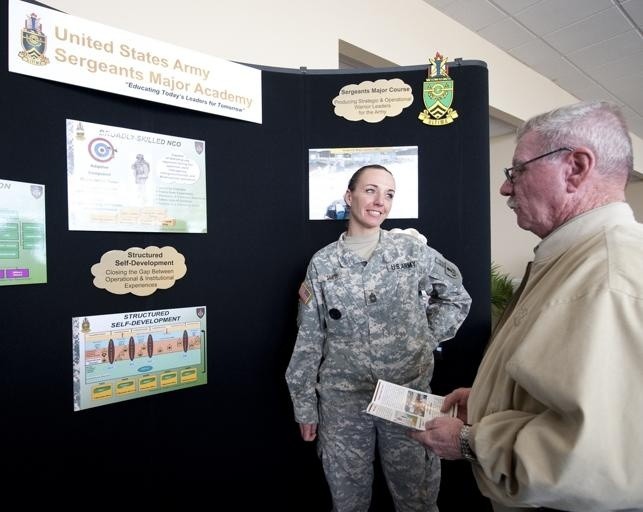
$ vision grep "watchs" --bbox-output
[459,424,476,463]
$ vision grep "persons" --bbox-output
[284,164,473,512]
[403,102,643,512]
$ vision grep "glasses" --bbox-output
[504,146,573,183]
